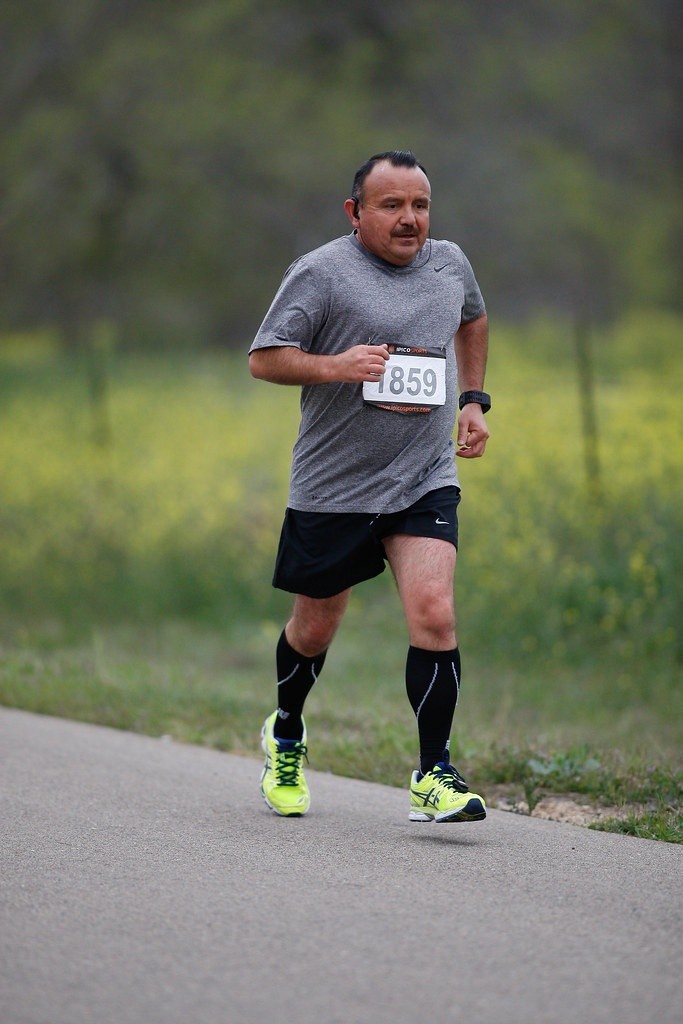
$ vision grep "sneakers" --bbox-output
[409,749,487,824]
[261,710,311,817]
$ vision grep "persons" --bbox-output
[245,151,491,828]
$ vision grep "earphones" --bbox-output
[351,196,360,219]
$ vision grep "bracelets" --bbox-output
[458,391,492,414]
